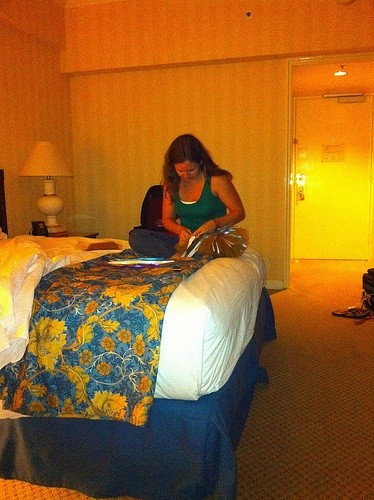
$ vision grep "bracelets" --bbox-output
[213,219,221,228]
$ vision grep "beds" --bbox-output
[0,169,277,500]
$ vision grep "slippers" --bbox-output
[333,306,354,315]
[347,309,370,318]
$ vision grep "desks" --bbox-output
[68,232,99,239]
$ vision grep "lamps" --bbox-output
[17,142,73,237]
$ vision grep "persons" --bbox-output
[129,134,248,261]
